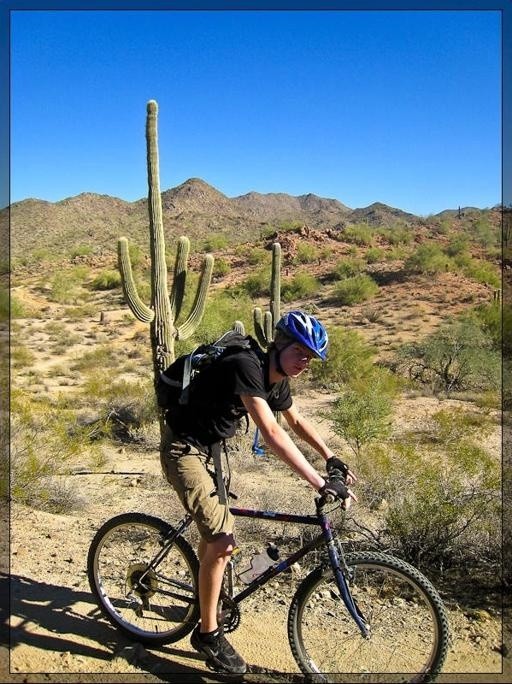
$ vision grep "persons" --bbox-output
[158,311,358,678]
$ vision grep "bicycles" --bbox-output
[87,459,452,684]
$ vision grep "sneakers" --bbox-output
[190,622,248,677]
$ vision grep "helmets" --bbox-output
[275,310,328,362]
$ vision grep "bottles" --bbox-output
[236,546,279,586]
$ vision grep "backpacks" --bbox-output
[154,332,266,413]
